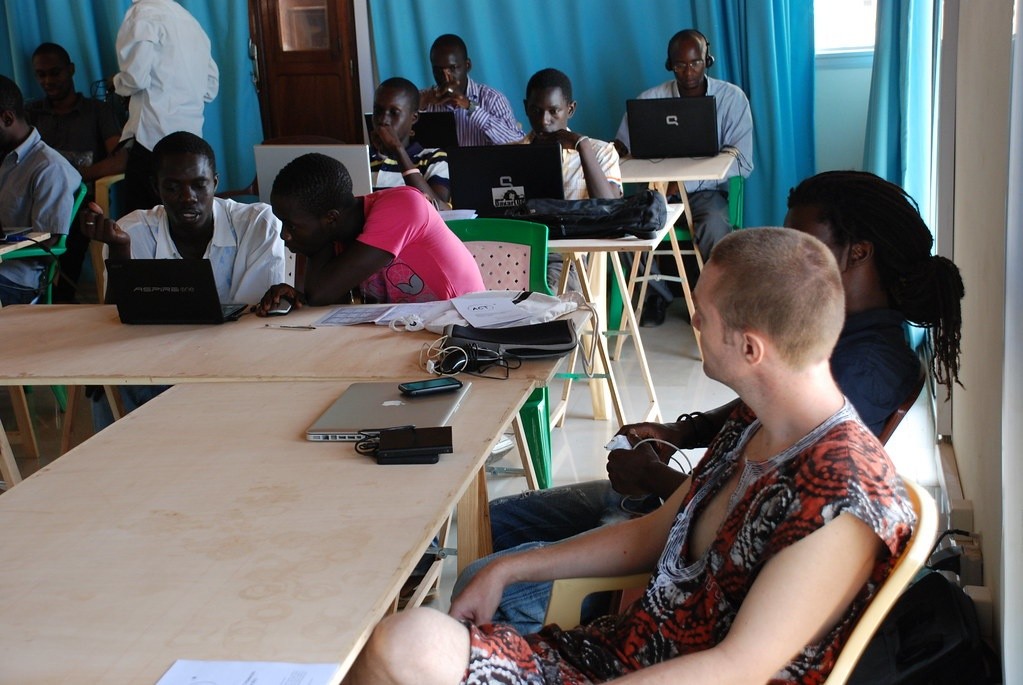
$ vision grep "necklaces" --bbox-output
[349,280,366,304]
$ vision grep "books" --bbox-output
[318,304,454,325]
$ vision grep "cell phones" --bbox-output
[398,377,464,396]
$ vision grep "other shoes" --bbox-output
[484,433,515,465]
[647,294,667,325]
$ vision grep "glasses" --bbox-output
[34,70,68,79]
[670,60,707,73]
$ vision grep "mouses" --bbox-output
[440,348,500,373]
[267,295,292,316]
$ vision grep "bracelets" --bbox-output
[402,169,420,176]
[677,413,699,449]
[690,411,714,441]
[575,136,588,150]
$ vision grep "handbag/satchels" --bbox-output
[844,572,1002,685]
[503,188,667,240]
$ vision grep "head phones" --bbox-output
[665,29,715,71]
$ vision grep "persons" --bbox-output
[24,42,133,294]
[0,75,92,305]
[342,227,917,685]
[399,170,965,638]
[419,33,525,146]
[79,132,296,304]
[614,29,755,327]
[516,69,624,295]
[257,153,486,315]
[371,78,452,210]
[107,0,219,214]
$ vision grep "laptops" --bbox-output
[365,110,460,156]
[305,381,472,441]
[253,143,372,204]
[626,95,722,158]
[0,220,34,243]
[105,259,247,325]
[449,142,565,219]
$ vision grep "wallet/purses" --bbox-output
[442,318,578,359]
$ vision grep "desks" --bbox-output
[0,138,742,685]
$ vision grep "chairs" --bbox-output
[607,171,744,331]
[443,215,558,492]
[0,179,90,414]
[89,172,127,303]
[878,345,927,446]
[540,476,940,685]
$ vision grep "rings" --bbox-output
[86,222,94,225]
[448,88,453,93]
